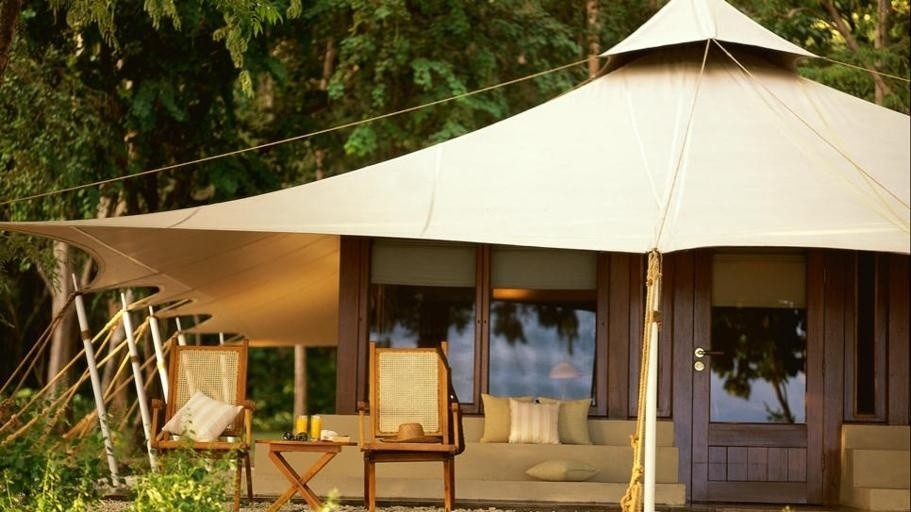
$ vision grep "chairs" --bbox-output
[359,336,464,512]
[147,334,255,510]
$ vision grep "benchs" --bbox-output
[835,422,911,511]
[236,395,687,508]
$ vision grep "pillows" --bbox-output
[560,395,596,446]
[509,398,560,442]
[162,389,243,442]
[524,458,600,483]
[478,393,510,442]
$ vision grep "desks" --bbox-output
[256,431,358,510]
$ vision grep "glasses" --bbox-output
[281,429,309,442]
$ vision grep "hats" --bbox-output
[379,421,441,444]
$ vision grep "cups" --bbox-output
[310,415,322,441]
[294,414,308,437]
